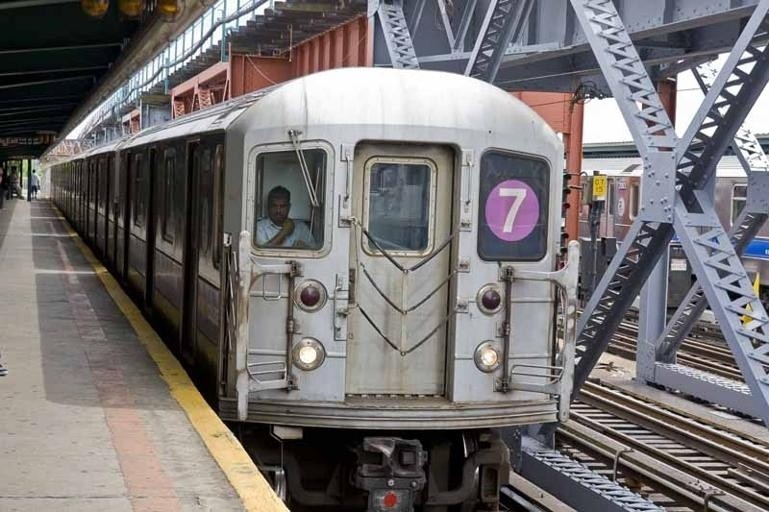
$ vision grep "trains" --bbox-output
[50,67,579,512]
[581,155,769,313]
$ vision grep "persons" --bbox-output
[252,184,318,251]
[31,169,43,201]
[9,165,25,199]
